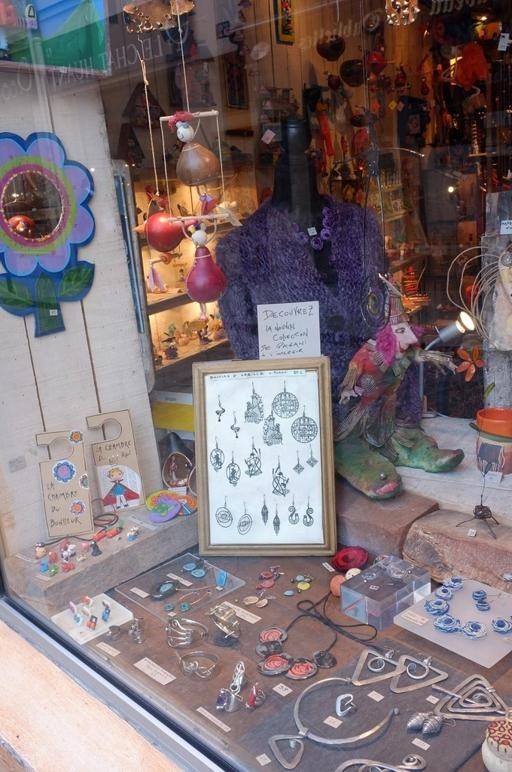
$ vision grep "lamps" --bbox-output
[412,311,477,416]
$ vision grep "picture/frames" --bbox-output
[191,356,338,558]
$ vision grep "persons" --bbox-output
[208,115,426,428]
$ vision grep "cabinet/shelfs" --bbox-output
[0,0,512,391]
